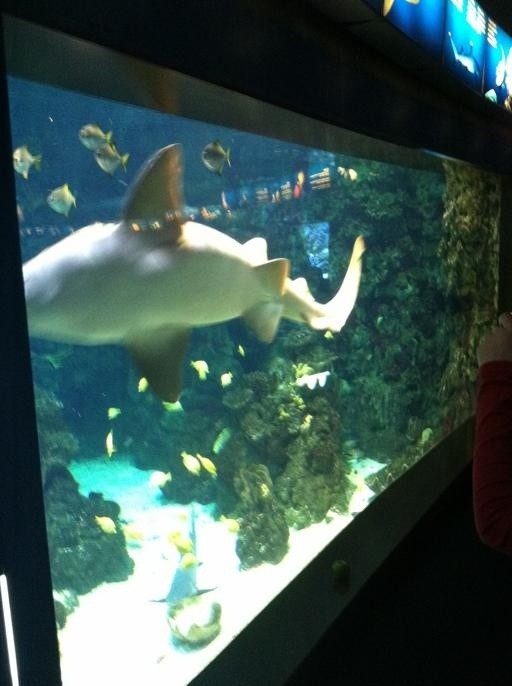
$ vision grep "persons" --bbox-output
[471,310,512,562]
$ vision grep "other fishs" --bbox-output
[10,119,367,655]
[420,426,434,446]
